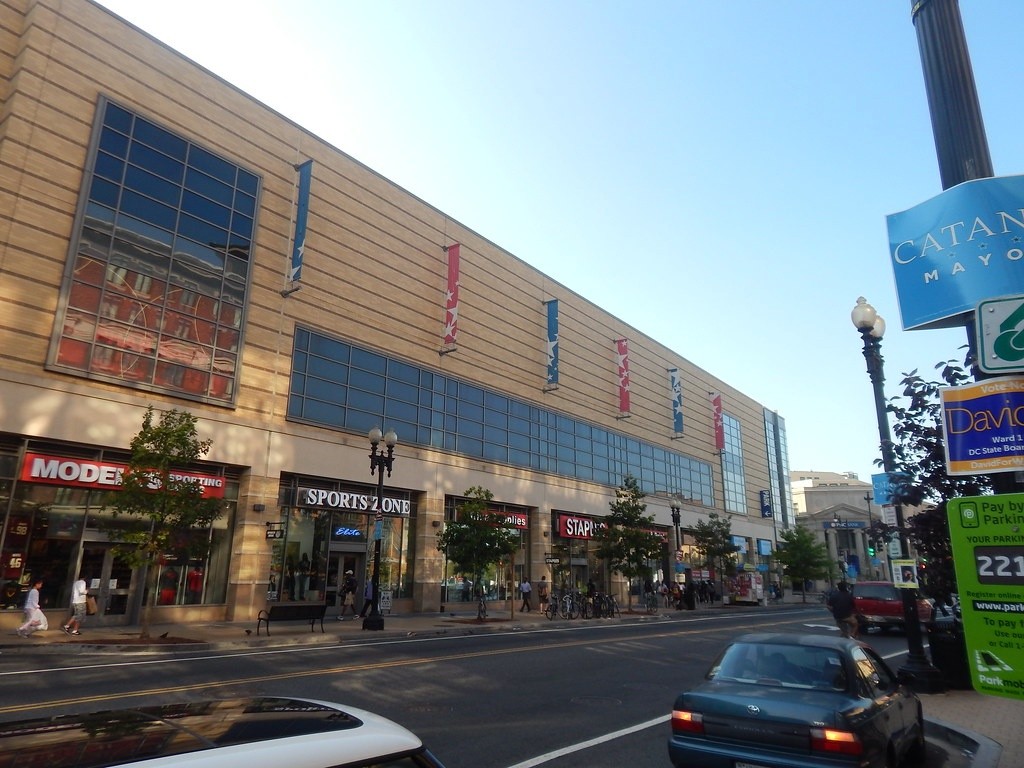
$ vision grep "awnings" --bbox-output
[737,562,769,572]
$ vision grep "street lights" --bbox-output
[849,294,945,694]
[360,422,398,632]
[668,494,682,550]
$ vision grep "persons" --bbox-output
[827,581,864,638]
[187,567,203,603]
[901,566,915,583]
[520,577,532,612]
[587,580,595,603]
[461,574,485,601]
[16,579,43,638]
[538,576,549,614]
[60,574,88,636]
[336,570,360,620]
[160,567,178,605]
[360,575,372,617]
[637,579,714,610]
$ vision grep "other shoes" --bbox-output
[352,615,361,620]
[336,616,344,621]
[71,631,82,636]
[360,614,367,618]
[16,627,30,639]
[527,608,531,612]
[540,610,547,615]
[60,626,71,636]
[848,634,856,640]
[520,610,523,612]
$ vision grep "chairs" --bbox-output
[767,652,806,683]
[820,659,845,687]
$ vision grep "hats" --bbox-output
[345,570,354,575]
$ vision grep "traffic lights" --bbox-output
[868,540,875,557]
[919,562,926,571]
[877,540,884,552]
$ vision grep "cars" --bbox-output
[0,693,450,768]
[851,579,935,636]
[665,629,926,768]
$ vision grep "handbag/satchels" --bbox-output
[34,606,48,631]
[86,591,98,615]
[344,591,354,606]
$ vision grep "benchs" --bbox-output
[257,605,327,637]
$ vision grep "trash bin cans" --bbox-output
[679,590,688,610]
[926,621,973,690]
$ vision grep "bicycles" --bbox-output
[647,593,660,615]
[473,593,489,622]
[544,590,622,621]
